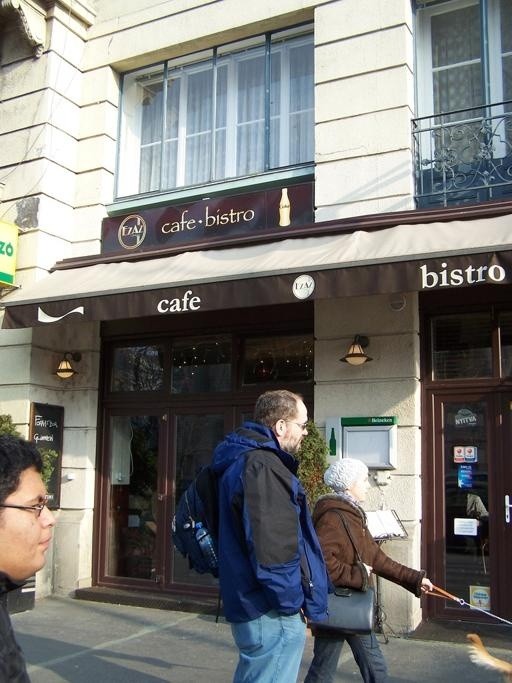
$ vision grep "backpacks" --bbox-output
[170,461,218,579]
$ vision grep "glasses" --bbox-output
[0,496,48,517]
[285,420,309,430]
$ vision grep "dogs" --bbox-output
[466,633,512,682]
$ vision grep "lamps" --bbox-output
[340,334,371,367]
[52,349,82,378]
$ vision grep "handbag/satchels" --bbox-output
[307,589,375,633]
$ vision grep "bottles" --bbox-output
[195,522,221,570]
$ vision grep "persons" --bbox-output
[303,458,434,682]
[213,389,329,682]
[1,432,57,683]
